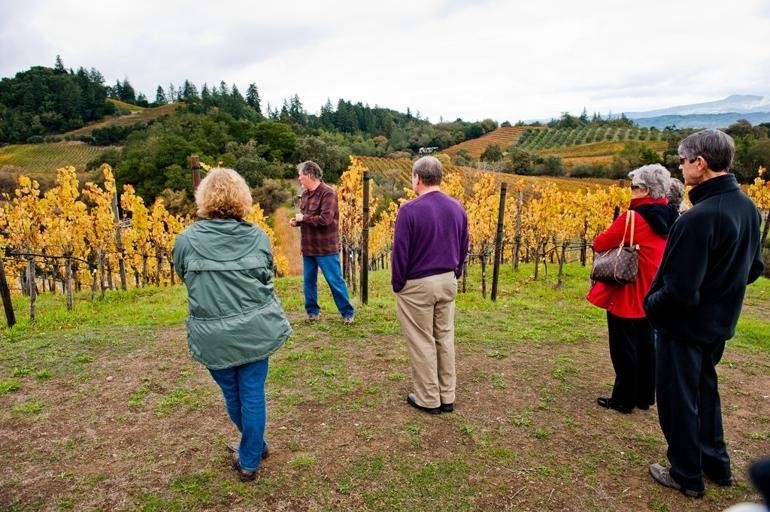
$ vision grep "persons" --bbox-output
[390,155,470,415]
[288,160,355,327]
[642,127,765,501]
[584,162,681,415]
[171,166,294,481]
[649,177,689,408]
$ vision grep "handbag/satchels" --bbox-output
[590,210,641,283]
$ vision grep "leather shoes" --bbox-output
[441,404,454,412]
[406,392,442,414]
[712,474,734,485]
[597,397,613,408]
[650,463,706,498]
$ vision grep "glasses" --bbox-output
[680,157,687,164]
[631,183,640,190]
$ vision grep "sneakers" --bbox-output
[310,314,319,321]
[231,451,257,481]
[343,314,355,325]
[227,440,270,458]
